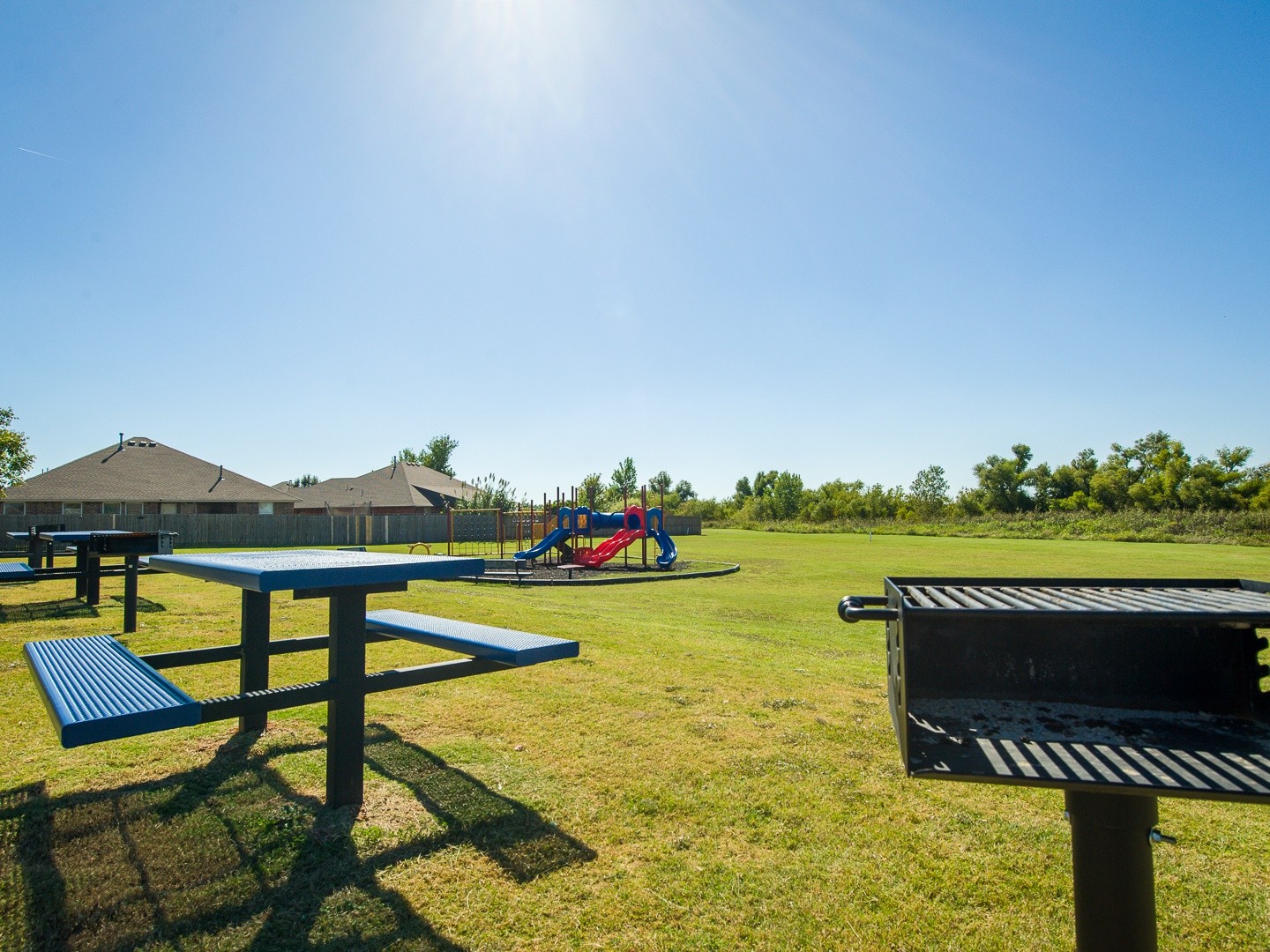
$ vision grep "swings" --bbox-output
[472,513,480,555]
[484,513,492,555]
[458,513,467,556]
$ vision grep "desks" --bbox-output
[148,548,487,810]
[39,530,134,604]
[6,531,36,568]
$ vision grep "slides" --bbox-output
[580,528,644,567]
[513,528,569,560]
[650,528,678,566]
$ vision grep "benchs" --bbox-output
[0,563,35,579]
[475,559,533,588]
[364,609,580,668]
[22,634,202,750]
[138,555,151,567]
[65,546,77,553]
